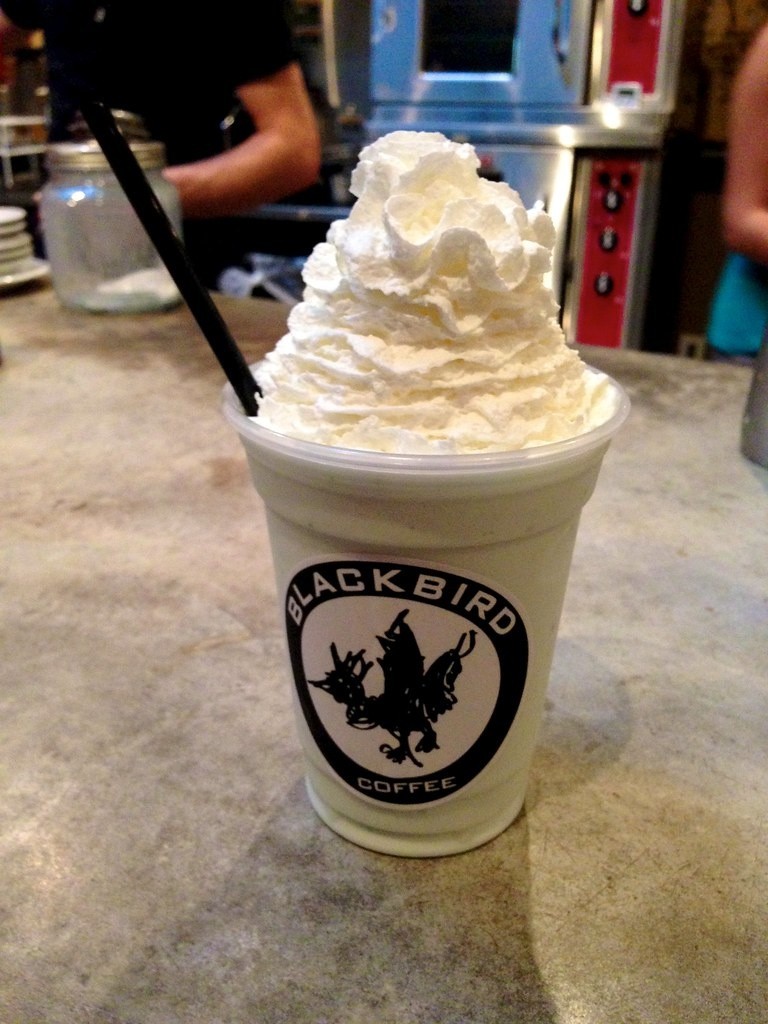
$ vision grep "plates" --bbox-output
[0,204,53,295]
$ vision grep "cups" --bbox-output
[218,368,628,859]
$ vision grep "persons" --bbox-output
[703,0,768,369]
[31,0,322,298]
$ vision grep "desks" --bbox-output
[1,278,767,1024]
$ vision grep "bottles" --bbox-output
[42,142,183,311]
